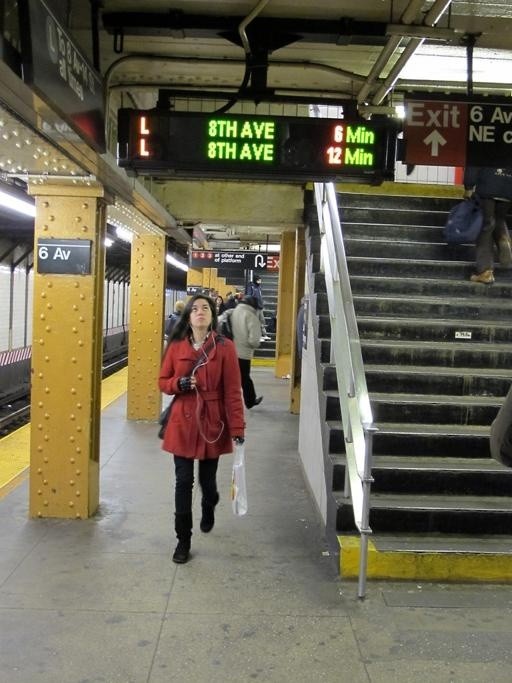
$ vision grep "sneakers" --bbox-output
[471,270,495,284]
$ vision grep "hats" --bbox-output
[239,295,262,309]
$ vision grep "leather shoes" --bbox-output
[248,396,262,408]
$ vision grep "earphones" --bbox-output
[188,320,191,325]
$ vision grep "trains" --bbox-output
[0,174,187,414]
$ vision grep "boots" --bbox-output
[173,513,192,563]
[201,495,218,531]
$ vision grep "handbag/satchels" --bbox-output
[232,447,249,516]
[159,405,171,439]
[445,203,482,242]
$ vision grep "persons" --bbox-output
[489,382,511,473]
[161,273,271,410]
[158,294,245,565]
[462,166,511,284]
